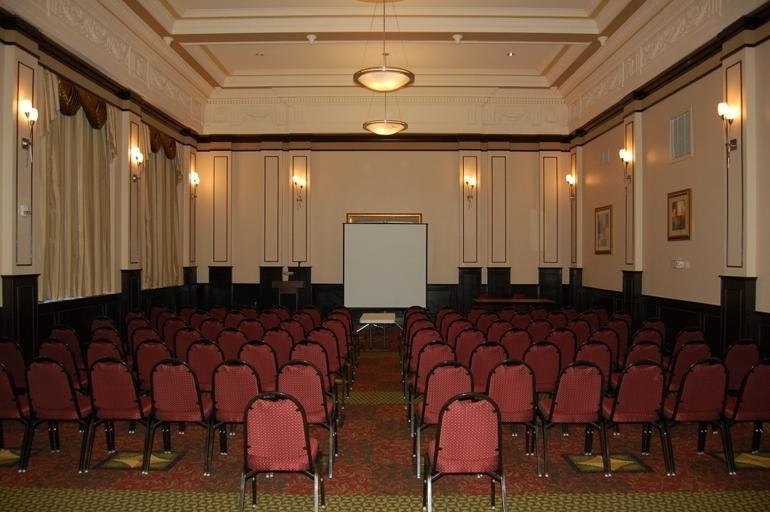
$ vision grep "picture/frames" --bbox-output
[595,205,613,255]
[667,188,692,241]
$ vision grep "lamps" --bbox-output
[191,172,200,200]
[292,176,306,201]
[717,102,738,151]
[363,53,408,137]
[133,148,144,182]
[353,1,415,94]
[465,176,477,201]
[619,149,632,183]
[566,174,576,199]
[22,99,38,149]
